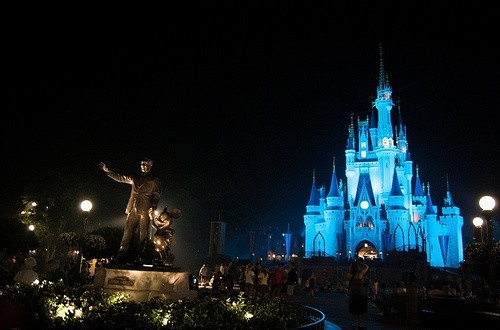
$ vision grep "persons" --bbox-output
[211,260,318,299]
[199,264,208,284]
[372,274,477,313]
[14,256,40,287]
[99,158,160,264]
[348,262,369,330]
[48,259,90,287]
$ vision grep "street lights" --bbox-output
[68,200,95,291]
[479,196,496,245]
[472,215,484,244]
